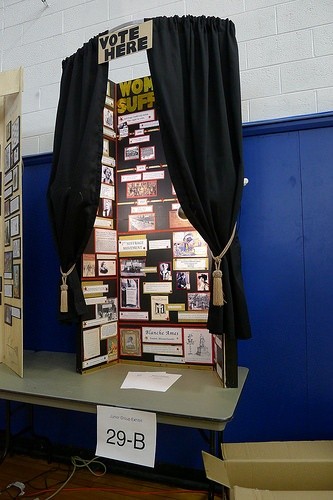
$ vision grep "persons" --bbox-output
[81,165,209,320]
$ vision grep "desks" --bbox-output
[0,350,249,500]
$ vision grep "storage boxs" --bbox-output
[202,440,333,500]
